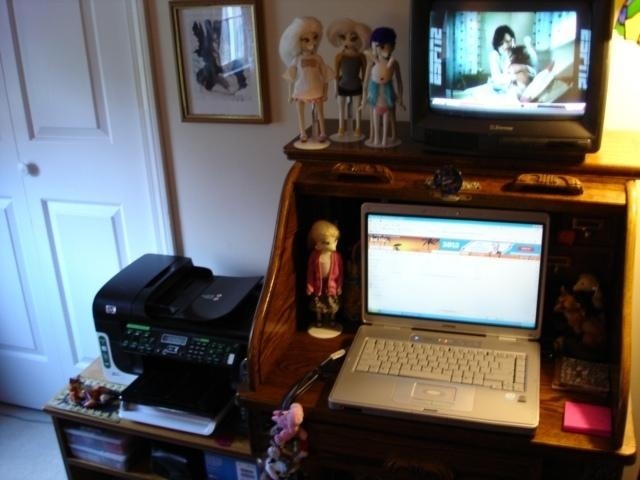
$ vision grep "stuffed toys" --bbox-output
[551,223,606,352]
[260,403,312,480]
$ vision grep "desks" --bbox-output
[244,117,639,478]
[42,353,252,480]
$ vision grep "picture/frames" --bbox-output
[168,0,272,124]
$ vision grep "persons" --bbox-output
[460,23,536,105]
[356,26,406,142]
[67,379,121,412]
[497,43,536,97]
[368,47,397,147]
[278,15,333,142]
[325,16,373,138]
[305,220,345,328]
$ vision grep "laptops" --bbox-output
[324,201,551,435]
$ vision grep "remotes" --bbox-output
[515,173,583,194]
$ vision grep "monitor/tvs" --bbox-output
[408,0,612,168]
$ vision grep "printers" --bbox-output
[91,253,267,385]
